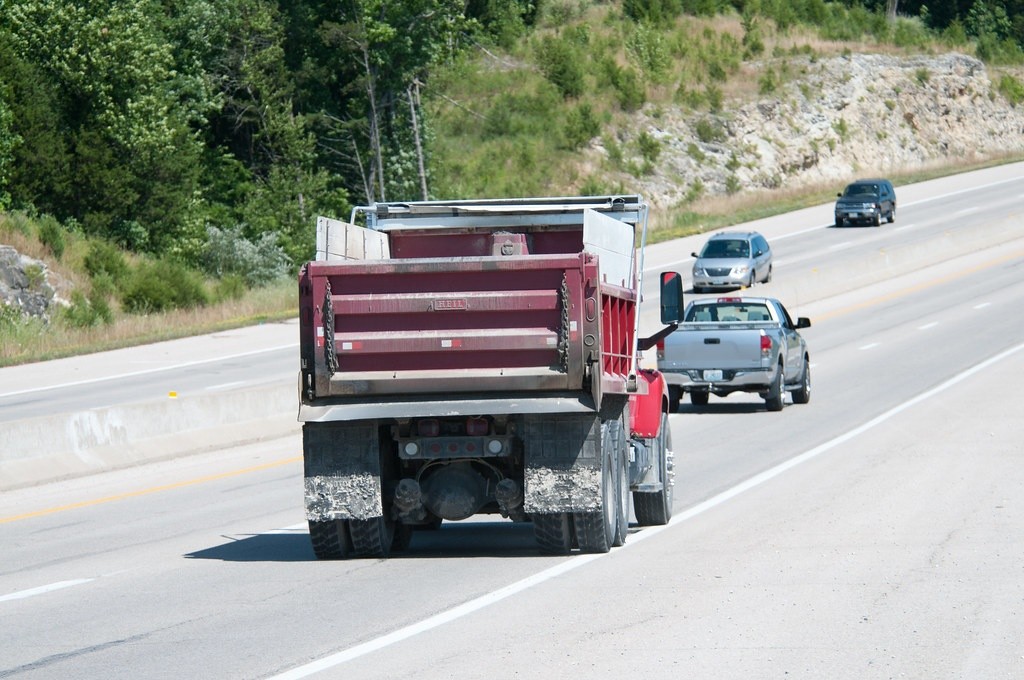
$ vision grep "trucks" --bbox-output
[297,187,685,560]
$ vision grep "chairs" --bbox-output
[748,312,764,320]
[696,312,711,321]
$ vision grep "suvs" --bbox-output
[836,179,897,228]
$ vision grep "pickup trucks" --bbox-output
[657,297,815,411]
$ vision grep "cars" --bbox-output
[692,230,773,292]
